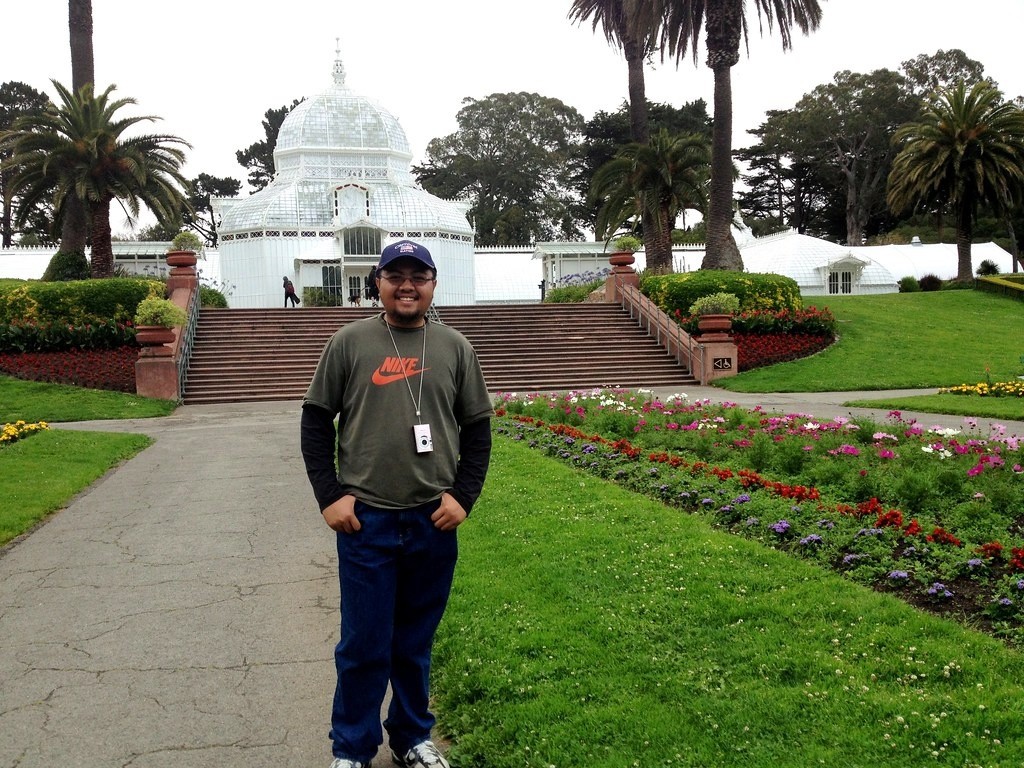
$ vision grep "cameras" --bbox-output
[413,423,434,453]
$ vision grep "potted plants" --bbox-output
[608,235,643,274]
[688,291,740,341]
[166,232,204,276]
[133,296,189,356]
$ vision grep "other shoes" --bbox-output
[372,302,378,307]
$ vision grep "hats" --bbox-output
[375,239,437,277]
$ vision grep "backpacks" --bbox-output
[286,284,294,296]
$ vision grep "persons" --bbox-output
[366,265,380,308]
[283,276,295,308]
[300,239,498,768]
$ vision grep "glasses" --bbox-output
[378,272,432,286]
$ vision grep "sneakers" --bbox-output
[391,741,450,768]
[328,757,372,768]
